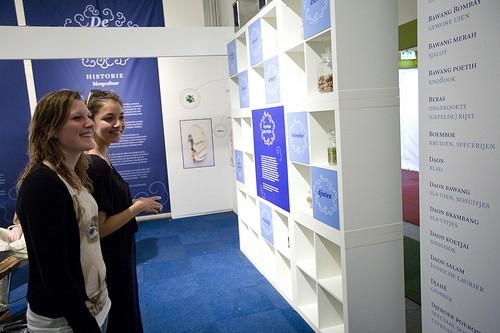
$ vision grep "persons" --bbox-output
[15,89,111,333]
[85,89,163,333]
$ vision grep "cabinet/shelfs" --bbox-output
[223,0,408,333]
